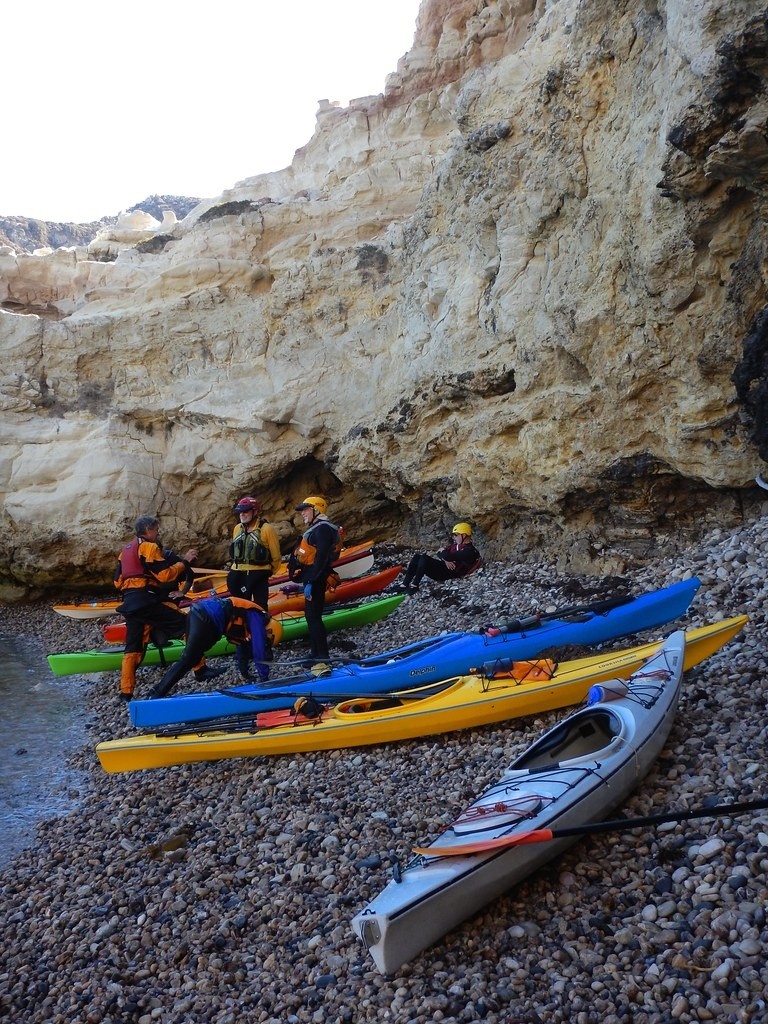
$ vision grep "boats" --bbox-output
[51,539,408,641]
[128,575,716,729]
[349,626,684,976]
[46,588,413,677]
[91,614,751,773]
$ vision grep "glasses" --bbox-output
[239,508,252,514]
[454,533,460,537]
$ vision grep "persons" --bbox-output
[113,515,229,704]
[225,497,282,683]
[389,522,483,598]
[287,496,345,679]
[147,596,284,703]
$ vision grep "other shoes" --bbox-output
[119,692,134,703]
[391,583,420,595]
[194,665,228,682]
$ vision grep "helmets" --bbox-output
[235,496,260,521]
[452,522,472,537]
[294,496,328,515]
[265,618,284,648]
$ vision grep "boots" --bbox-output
[148,671,180,699]
[287,632,332,668]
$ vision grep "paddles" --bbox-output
[256,657,376,667]
[412,798,767,857]
[213,688,438,702]
